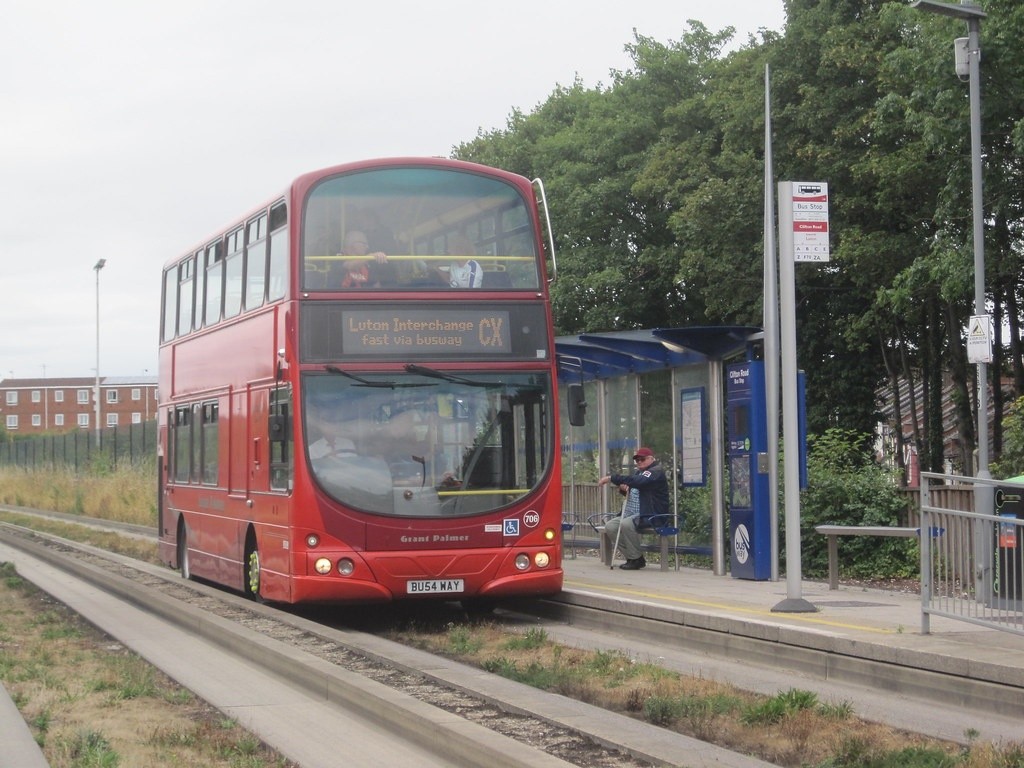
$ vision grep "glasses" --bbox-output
[636,457,651,463]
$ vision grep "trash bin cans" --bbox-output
[996,485,1024,600]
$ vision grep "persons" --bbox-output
[307,407,455,486]
[598,448,670,570]
[327,229,481,290]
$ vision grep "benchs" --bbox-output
[561,512,579,560]
[814,524,944,600]
[586,512,686,572]
[480,263,513,288]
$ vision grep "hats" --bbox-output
[632,447,654,459]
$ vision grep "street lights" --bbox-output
[909,0,994,608]
[92,260,106,453]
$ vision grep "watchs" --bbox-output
[608,475,612,482]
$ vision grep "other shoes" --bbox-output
[620,557,646,569]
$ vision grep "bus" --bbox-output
[156,157,588,614]
